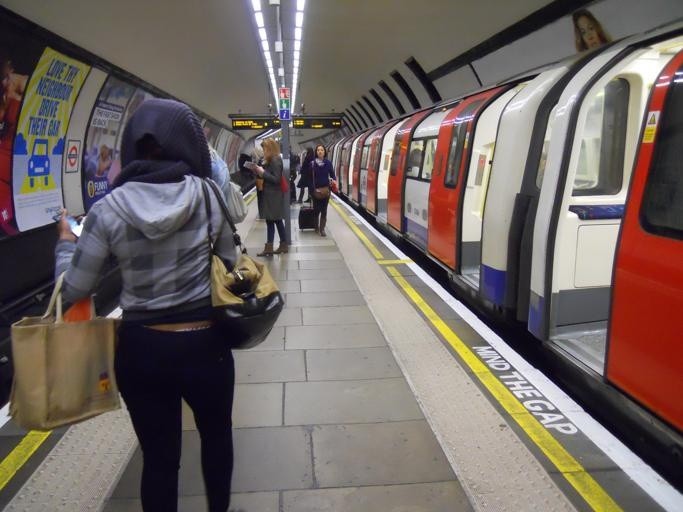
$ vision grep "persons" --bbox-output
[206,139,230,196]
[54,99,234,511]
[253,138,336,256]
[573,8,611,53]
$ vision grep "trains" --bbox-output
[325,13,683,481]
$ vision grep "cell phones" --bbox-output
[51,211,83,237]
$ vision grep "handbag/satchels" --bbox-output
[281,175,289,193]
[313,187,329,200]
[201,177,284,349]
[7,271,122,432]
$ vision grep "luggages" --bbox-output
[299,201,315,232]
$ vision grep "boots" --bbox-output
[314,215,318,233]
[320,217,326,235]
[256,242,273,257]
[273,242,288,254]
